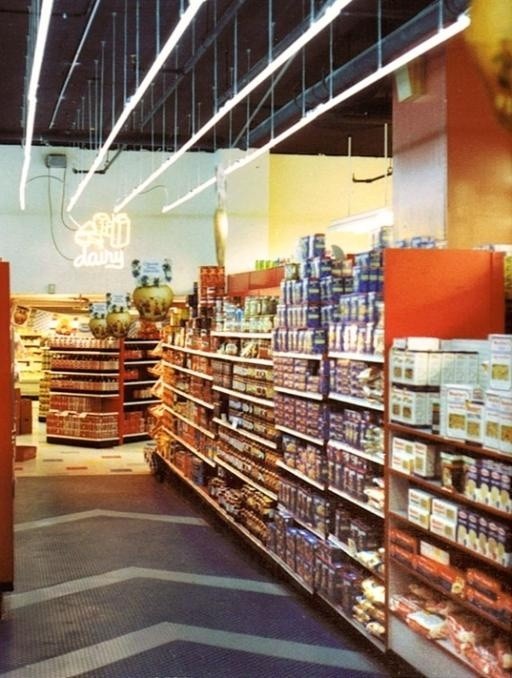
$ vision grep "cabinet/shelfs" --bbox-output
[46,336,122,445]
[213,326,277,548]
[269,350,324,605]
[317,349,387,656]
[154,330,213,509]
[122,336,160,448]
[387,335,512,678]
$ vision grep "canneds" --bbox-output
[270,224,392,354]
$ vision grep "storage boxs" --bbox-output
[13,389,21,435]
[20,399,32,433]
